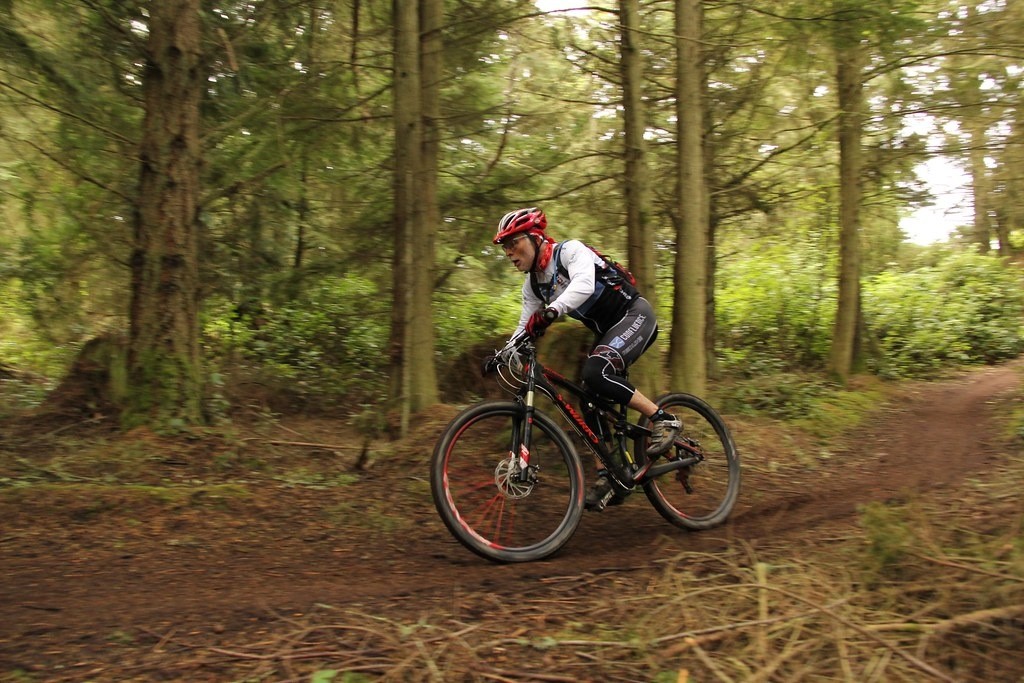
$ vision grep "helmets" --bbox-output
[493,207,547,244]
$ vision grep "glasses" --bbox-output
[502,235,528,251]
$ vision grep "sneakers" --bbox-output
[646,414,684,454]
[585,477,611,504]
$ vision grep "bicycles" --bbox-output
[430,307,741,563]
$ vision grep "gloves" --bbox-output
[525,311,544,336]
[482,356,500,376]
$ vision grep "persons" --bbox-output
[480,206,684,509]
